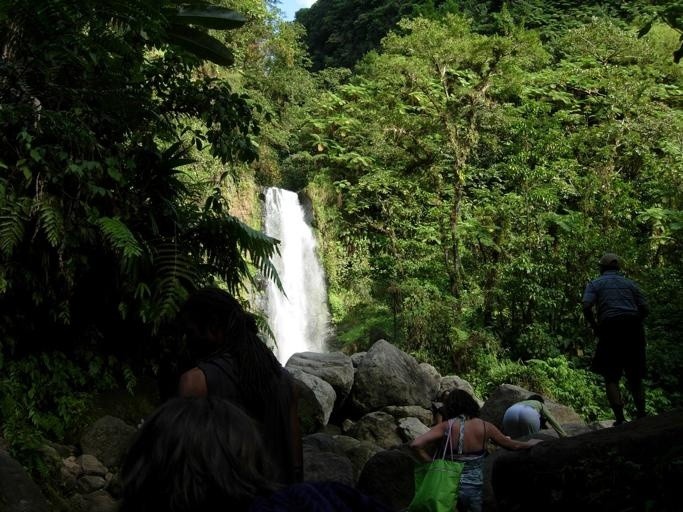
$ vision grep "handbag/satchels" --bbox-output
[407,460,465,511]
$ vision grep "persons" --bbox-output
[410,389,543,512]
[178,285,305,486]
[101,392,388,511]
[498,394,569,441]
[582,254,651,427]
